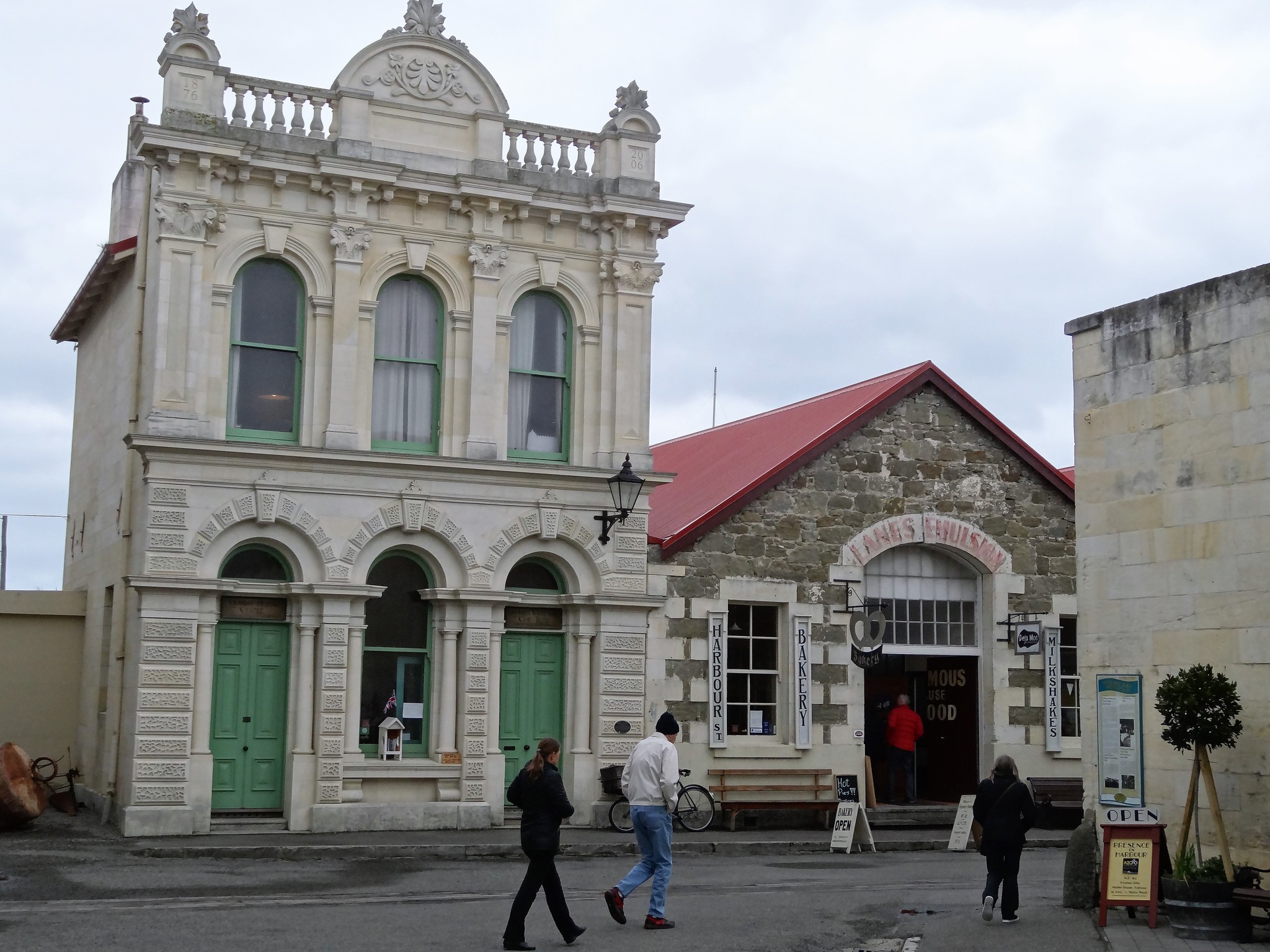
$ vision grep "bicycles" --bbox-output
[608,768,715,833]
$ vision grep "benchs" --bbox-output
[707,769,839,832]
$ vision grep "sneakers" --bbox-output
[1002,915,1019,922]
[981,896,993,921]
[643,914,675,929]
[604,888,628,925]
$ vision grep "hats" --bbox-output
[656,712,680,734]
[898,695,909,705]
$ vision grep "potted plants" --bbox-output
[1154,662,1258,942]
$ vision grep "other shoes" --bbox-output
[503,940,536,951]
[565,927,586,944]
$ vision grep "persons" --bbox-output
[604,712,679,929]
[972,754,1036,923]
[884,694,924,804]
[502,737,587,951]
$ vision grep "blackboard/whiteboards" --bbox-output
[834,773,861,803]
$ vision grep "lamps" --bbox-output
[593,453,646,545]
[728,618,742,630]
[259,385,290,401]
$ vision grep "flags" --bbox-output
[383,691,396,714]
